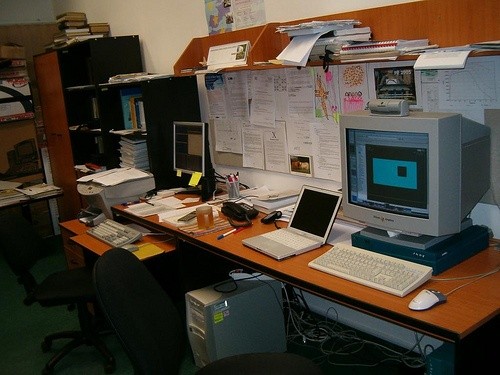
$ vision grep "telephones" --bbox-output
[221,202,259,226]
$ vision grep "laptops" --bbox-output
[240,182,344,263]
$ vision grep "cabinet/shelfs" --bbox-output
[168,0,500,78]
[32,35,143,270]
[0,44,45,183]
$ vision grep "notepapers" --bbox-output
[177,170,181,177]
[188,171,203,187]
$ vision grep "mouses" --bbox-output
[407,287,448,314]
[120,243,141,253]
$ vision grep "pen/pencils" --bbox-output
[225,172,240,198]
[218,227,236,240]
[234,227,247,234]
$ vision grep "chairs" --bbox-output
[0,206,119,374]
[93,248,320,374]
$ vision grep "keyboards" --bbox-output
[85,217,142,250]
[307,240,435,299]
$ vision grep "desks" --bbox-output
[57,183,500,342]
[0,182,65,206]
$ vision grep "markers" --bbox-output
[122,199,143,205]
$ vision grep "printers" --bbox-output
[76,165,156,227]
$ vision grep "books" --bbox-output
[340,39,440,61]
[253,190,300,214]
[46,11,103,49]
[275,21,371,61]
[119,89,148,132]
[89,21,110,37]
[85,163,103,172]
[0,183,63,208]
[118,137,150,172]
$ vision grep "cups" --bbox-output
[196,205,214,230]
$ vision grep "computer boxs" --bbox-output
[350,223,490,277]
[423,341,454,375]
[184,270,291,369]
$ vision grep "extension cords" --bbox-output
[228,267,277,282]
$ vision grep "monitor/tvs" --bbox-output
[171,118,224,204]
[337,107,493,252]
